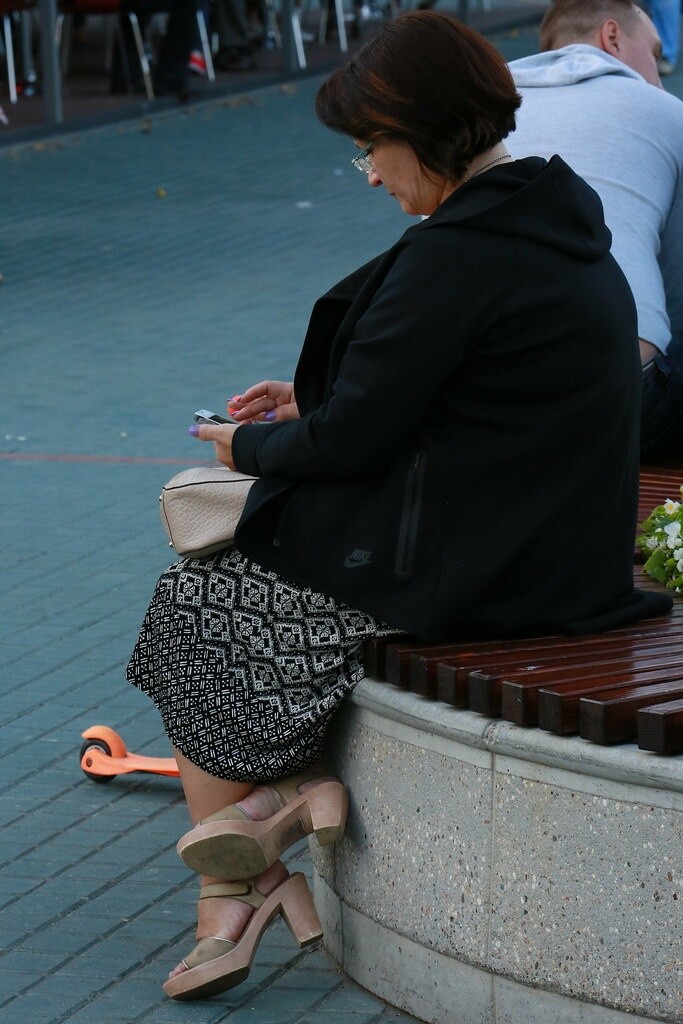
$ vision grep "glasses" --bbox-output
[351,142,373,174]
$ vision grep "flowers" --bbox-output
[635,485,683,593]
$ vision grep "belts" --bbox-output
[642,351,672,384]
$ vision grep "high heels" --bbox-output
[176,769,350,881]
[162,871,324,1001]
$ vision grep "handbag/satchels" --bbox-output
[158,466,260,559]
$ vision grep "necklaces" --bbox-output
[464,155,512,182]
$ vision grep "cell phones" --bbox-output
[193,409,238,425]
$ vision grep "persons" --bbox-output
[110,1,268,96]
[503,0,683,467]
[126,9,673,998]
[314,0,363,41]
[640,0,680,72]
